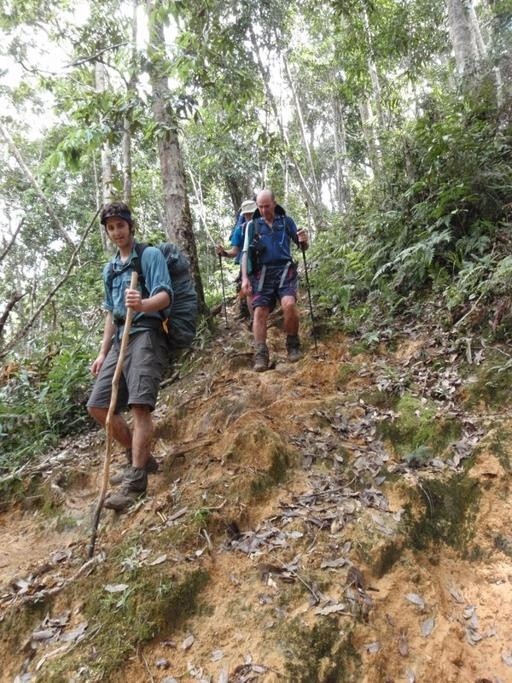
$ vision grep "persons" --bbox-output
[85,200,174,511]
[214,199,258,326]
[240,189,310,373]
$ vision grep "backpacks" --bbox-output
[106,242,199,350]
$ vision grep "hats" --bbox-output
[239,200,258,217]
[252,204,286,220]
[100,209,132,226]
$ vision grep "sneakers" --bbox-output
[233,311,249,320]
[103,467,148,510]
[108,445,159,486]
[285,334,304,363]
[253,343,269,372]
